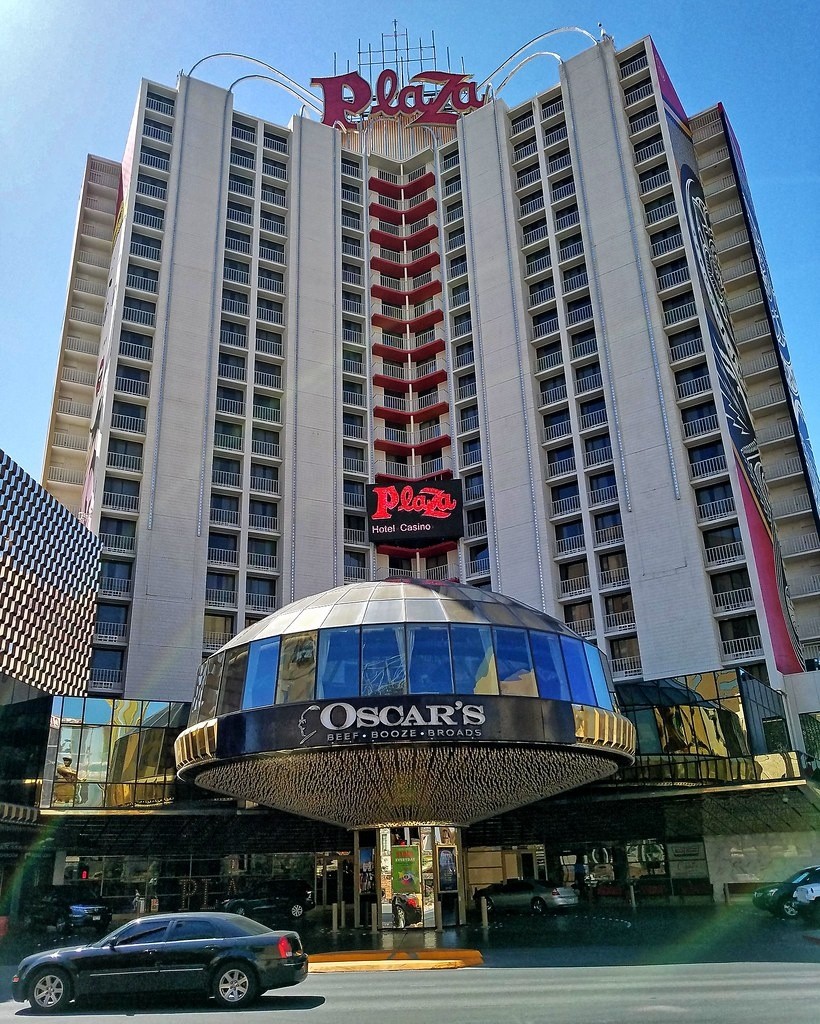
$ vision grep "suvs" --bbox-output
[18,884,113,936]
[220,878,317,923]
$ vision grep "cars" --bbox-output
[473,878,579,918]
[752,864,820,920]
[12,912,309,1016]
